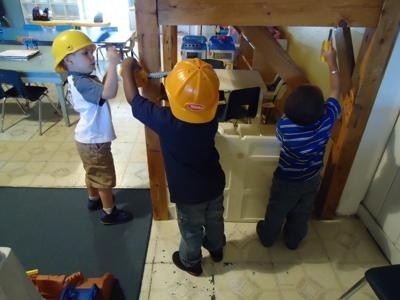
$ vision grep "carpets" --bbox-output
[0,186,153,300]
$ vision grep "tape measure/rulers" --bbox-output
[319,29,332,62]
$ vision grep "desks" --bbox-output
[0,45,73,127]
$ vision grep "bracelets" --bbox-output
[330,69,339,74]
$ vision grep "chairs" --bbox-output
[204,59,287,123]
[337,263,399,300]
[0,69,58,136]
[0,23,137,65]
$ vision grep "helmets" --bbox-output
[164,58,220,124]
[52,29,96,73]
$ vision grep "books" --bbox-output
[0,49,40,62]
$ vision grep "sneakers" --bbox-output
[257,220,272,247]
[283,221,298,250]
[87,193,116,211]
[172,251,202,276]
[99,205,132,225]
[202,234,223,262]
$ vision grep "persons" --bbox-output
[52,29,133,225]
[121,58,226,276]
[257,38,342,249]
